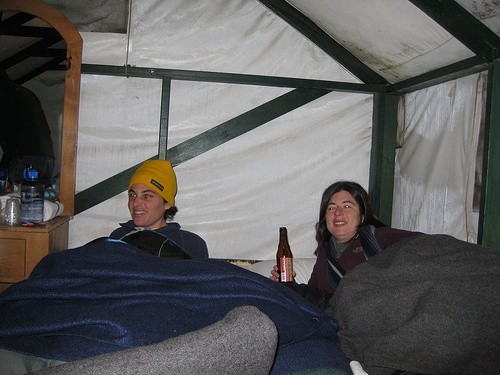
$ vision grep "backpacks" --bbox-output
[0,67,56,189]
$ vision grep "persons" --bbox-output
[271,180,428,311]
[0,66,56,189]
[107,159,210,259]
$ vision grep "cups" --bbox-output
[4,198,20,225]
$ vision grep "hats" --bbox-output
[128,159,177,208]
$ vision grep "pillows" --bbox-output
[246,257,316,285]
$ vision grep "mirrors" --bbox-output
[0,0,83,219]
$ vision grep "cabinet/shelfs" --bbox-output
[0,216,70,284]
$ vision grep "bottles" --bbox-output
[277,227,294,284]
[21,168,44,223]
